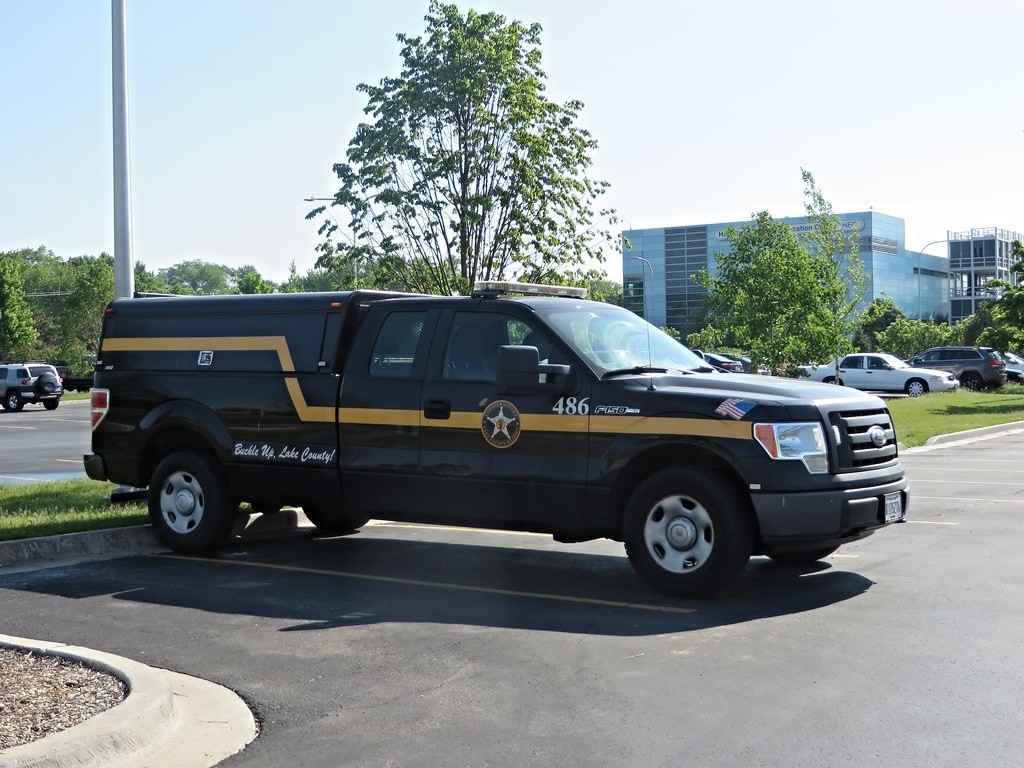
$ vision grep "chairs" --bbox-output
[522,333,549,362]
[459,318,508,370]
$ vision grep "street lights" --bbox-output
[916,239,962,326]
[630,255,657,327]
[302,196,358,288]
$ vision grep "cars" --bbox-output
[581,349,788,378]
[997,349,1024,382]
[799,352,961,397]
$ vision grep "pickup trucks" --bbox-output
[81,279,912,599]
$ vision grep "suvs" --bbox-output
[899,345,1008,391]
[0,362,65,415]
